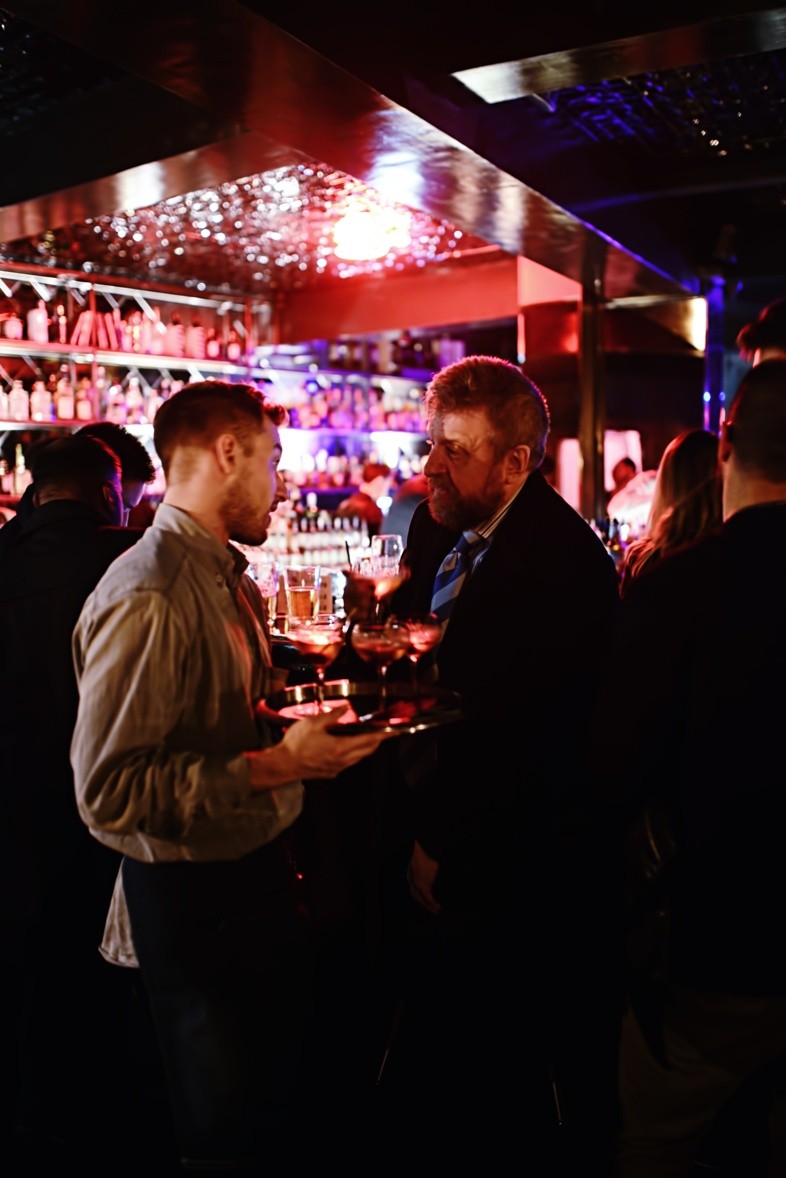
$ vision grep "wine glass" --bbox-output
[290,613,345,715]
[352,622,412,728]
[386,613,442,727]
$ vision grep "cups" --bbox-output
[247,565,322,634]
[370,531,403,582]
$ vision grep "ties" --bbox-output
[429,532,490,680]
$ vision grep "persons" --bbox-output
[615,427,725,588]
[380,435,437,557]
[74,423,159,530]
[605,456,637,504]
[336,459,395,535]
[66,379,394,1177]
[0,434,153,1178]
[608,352,786,1177]
[324,349,634,1178]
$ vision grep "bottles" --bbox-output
[608,518,624,562]
[235,512,369,569]
[1,293,204,359]
[0,374,185,423]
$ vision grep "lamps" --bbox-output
[332,206,395,262]
